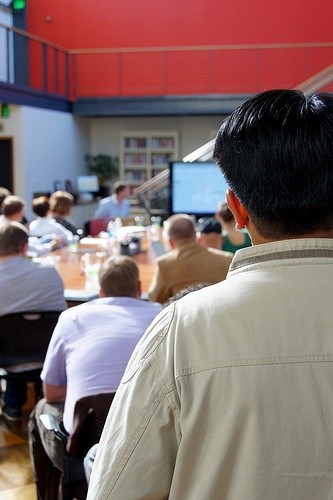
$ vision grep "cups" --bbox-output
[80,251,103,280]
[67,234,80,252]
[99,215,163,242]
[45,255,61,269]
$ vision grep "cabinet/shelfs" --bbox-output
[119,130,181,188]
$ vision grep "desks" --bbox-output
[28,222,176,308]
[64,198,100,227]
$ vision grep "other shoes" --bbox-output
[2,405,22,421]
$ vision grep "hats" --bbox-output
[199,218,222,234]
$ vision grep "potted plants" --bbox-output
[85,153,119,187]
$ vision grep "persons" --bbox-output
[89,180,131,221]
[195,218,224,252]
[214,200,253,255]
[0,194,64,259]
[0,219,68,424]
[46,190,83,241]
[84,89,333,500]
[25,253,164,500]
[29,195,80,249]
[0,187,30,231]
[145,213,234,305]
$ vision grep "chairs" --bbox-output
[0,309,63,409]
[39,390,118,500]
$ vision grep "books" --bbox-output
[150,153,174,165]
[122,153,147,165]
[151,169,163,178]
[124,170,146,183]
[151,138,174,149]
[122,136,147,150]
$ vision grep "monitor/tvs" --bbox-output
[170,161,230,219]
